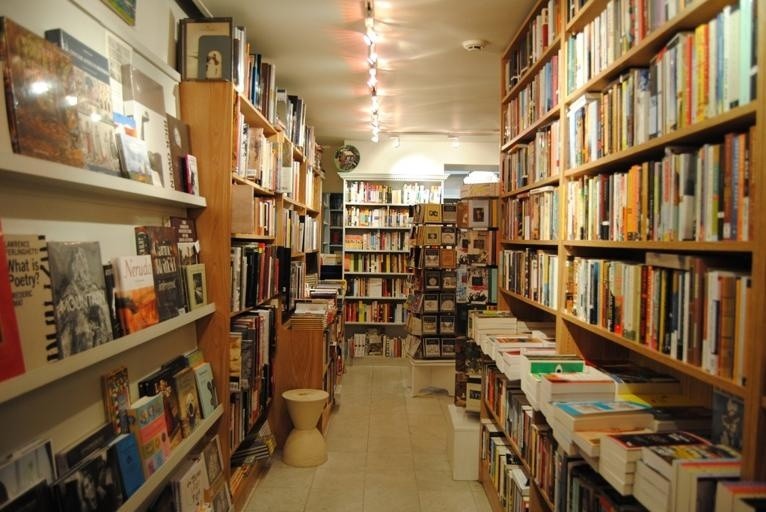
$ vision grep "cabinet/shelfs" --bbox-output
[1,2,232,510]
[447,0,764,508]
[280,278,346,431]
[179,17,323,512]
[324,169,454,398]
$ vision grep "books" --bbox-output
[0,0,343,511]
[459,0,765,510]
[344,176,457,361]
